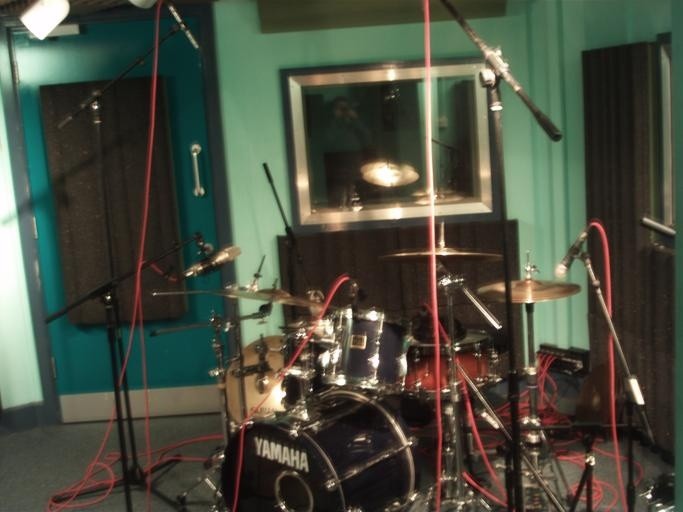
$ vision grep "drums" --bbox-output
[404,330,494,400]
[281,334,338,410]
[224,337,291,424]
[222,386,419,507]
[370,389,454,496]
[321,306,414,396]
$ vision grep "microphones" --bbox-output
[554,223,593,278]
[166,4,200,50]
[177,243,241,279]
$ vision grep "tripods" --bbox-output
[52,23,189,512]
[383,260,637,512]
[150,302,273,512]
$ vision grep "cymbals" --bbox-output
[209,286,326,310]
[478,281,579,302]
[384,248,502,263]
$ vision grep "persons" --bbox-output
[446,79,476,189]
[310,96,369,203]
